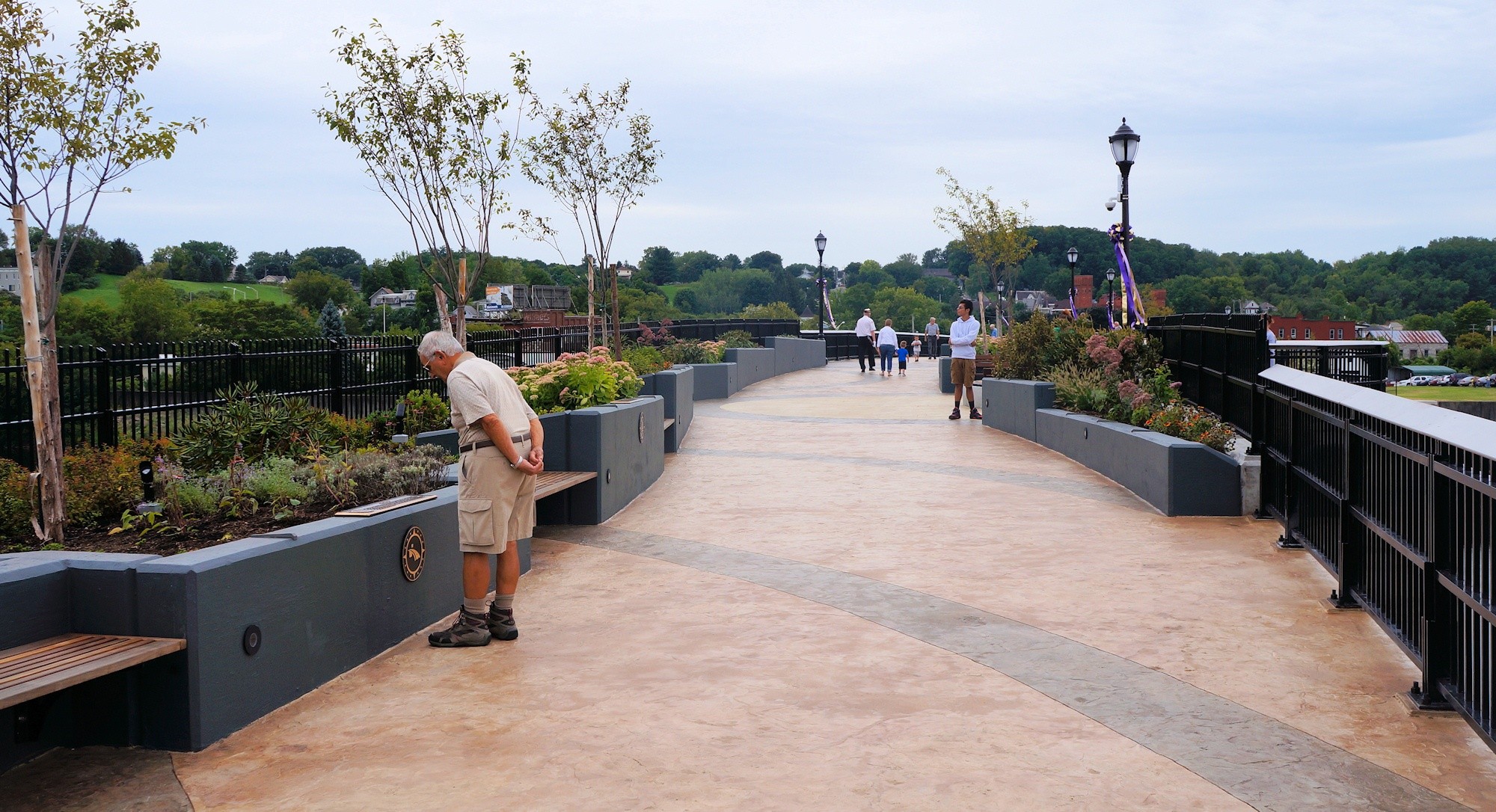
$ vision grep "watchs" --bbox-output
[510,454,524,471]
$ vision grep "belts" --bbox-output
[460,432,531,453]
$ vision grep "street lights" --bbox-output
[997,280,1005,316]
[1105,118,1141,331]
[1066,246,1078,316]
[814,230,827,340]
[1106,266,1116,331]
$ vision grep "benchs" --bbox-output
[0,633,188,747]
[975,355,1010,380]
[664,418,675,430]
[535,471,598,525]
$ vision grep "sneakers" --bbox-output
[428,603,490,647]
[485,600,519,641]
[970,408,982,419]
[949,407,961,419]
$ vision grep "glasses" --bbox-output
[958,306,966,309]
[422,350,444,371]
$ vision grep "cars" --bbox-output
[1386,373,1496,387]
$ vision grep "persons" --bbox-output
[989,323,998,337]
[924,317,940,359]
[896,340,909,377]
[911,335,922,362]
[1471,376,1478,388]
[1485,374,1491,388]
[876,318,899,376]
[1113,321,1122,331]
[854,308,876,372]
[1262,314,1277,367]
[417,329,545,650]
[948,299,982,420]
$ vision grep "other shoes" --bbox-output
[888,374,892,376]
[934,356,936,359]
[902,374,906,376]
[880,373,884,375]
[869,368,875,371]
[899,373,901,375]
[862,370,865,373]
[929,356,931,359]
